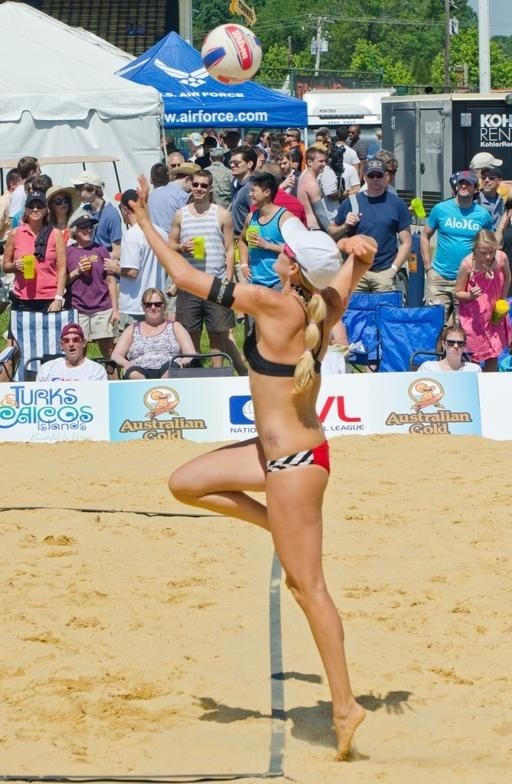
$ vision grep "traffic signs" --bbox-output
[228,0,258,27]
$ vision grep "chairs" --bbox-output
[371,299,444,373]
[169,351,235,377]
[339,288,404,373]
[405,348,474,373]
[20,351,106,383]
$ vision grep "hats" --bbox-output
[181,132,204,146]
[70,171,105,188]
[456,171,479,186]
[281,215,344,290]
[166,162,202,176]
[61,324,83,339]
[469,152,504,169]
[481,165,502,176]
[364,157,386,175]
[45,185,76,202]
[69,214,99,228]
[24,191,47,206]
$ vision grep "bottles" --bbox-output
[490,299,509,326]
[233,238,241,262]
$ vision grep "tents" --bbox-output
[0,2,164,236]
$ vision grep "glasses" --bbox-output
[385,167,397,174]
[229,160,245,165]
[443,340,465,347]
[62,337,83,343]
[51,198,69,206]
[192,182,210,189]
[143,301,164,308]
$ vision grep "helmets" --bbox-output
[209,147,223,157]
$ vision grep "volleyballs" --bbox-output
[201,23,262,86]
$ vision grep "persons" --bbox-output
[128,175,379,761]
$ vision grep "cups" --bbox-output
[191,236,205,260]
[246,226,261,247]
[411,197,426,218]
[21,255,36,281]
[79,254,93,271]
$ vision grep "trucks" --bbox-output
[300,85,396,172]
